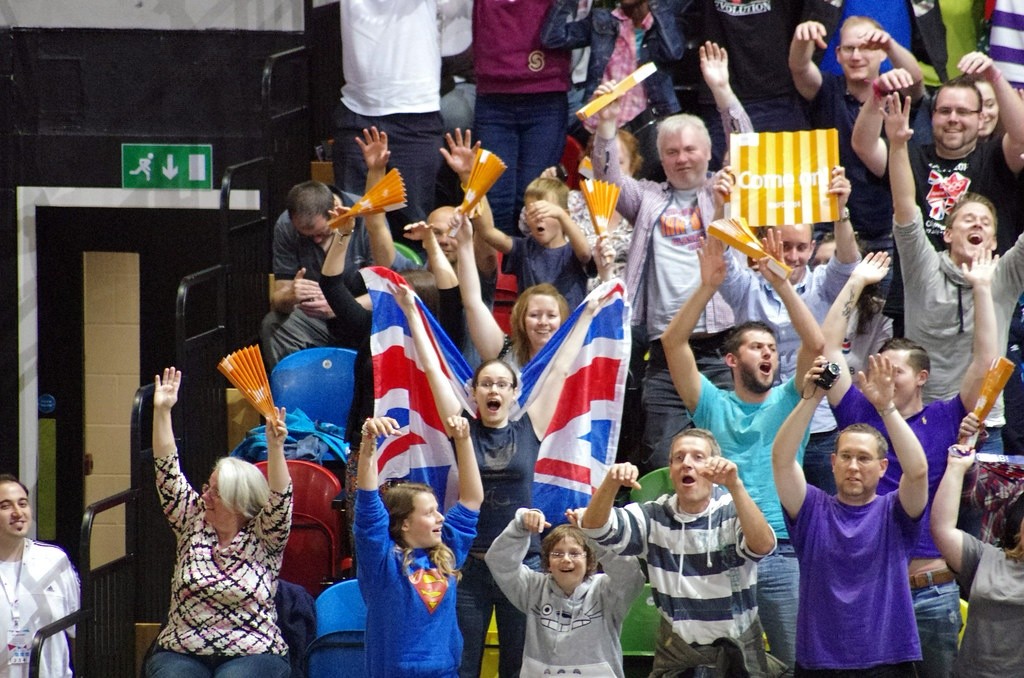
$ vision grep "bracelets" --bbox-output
[877,406,896,416]
[361,418,377,439]
[988,70,1002,81]
[460,183,467,193]
[335,229,354,237]
[360,441,379,454]
[864,79,888,100]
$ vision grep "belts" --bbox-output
[904,572,955,591]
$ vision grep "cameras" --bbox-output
[814,362,842,390]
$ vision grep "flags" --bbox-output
[359,265,632,532]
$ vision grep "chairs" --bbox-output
[560,135,584,190]
[481,605,500,678]
[619,583,660,656]
[492,251,518,336]
[252,347,367,678]
[629,467,675,504]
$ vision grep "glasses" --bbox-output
[933,103,982,120]
[832,452,882,468]
[200,483,221,499]
[838,42,881,60]
[549,550,586,560]
[475,379,513,391]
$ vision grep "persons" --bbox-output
[929,444,1024,678]
[145,366,295,678]
[577,428,778,678]
[485,507,647,678]
[260,0,1024,678]
[772,350,930,678]
[352,414,483,678]
[0,474,82,678]
